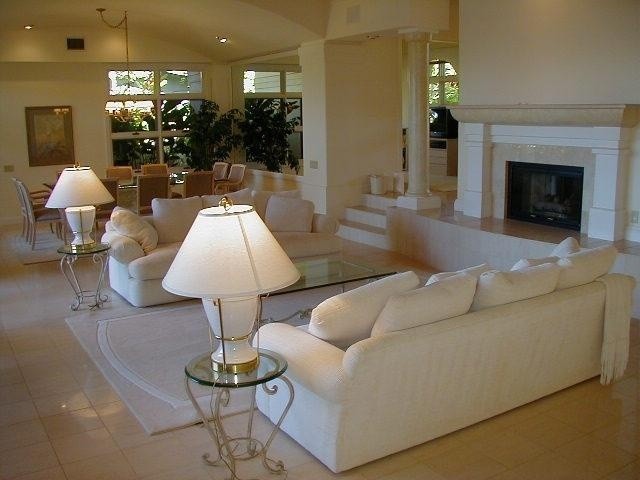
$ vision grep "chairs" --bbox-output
[215,163,247,195]
[17,180,68,250]
[130,174,170,217]
[10,177,64,251]
[177,171,216,199]
[213,162,231,181]
[104,165,133,185]
[94,177,119,231]
[140,163,170,175]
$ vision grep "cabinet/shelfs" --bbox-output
[429,138,457,178]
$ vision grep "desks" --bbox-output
[97,182,183,218]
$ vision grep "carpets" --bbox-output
[64,255,436,437]
[404,171,458,192]
[3,219,107,265]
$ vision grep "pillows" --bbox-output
[549,237,582,260]
[252,189,303,222]
[201,187,253,209]
[510,255,561,271]
[425,262,496,288]
[265,195,315,233]
[110,206,158,253]
[199,187,252,205]
[470,263,559,314]
[556,245,618,293]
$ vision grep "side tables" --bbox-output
[183,346,295,480]
[56,244,115,311]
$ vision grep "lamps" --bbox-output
[96,6,157,136]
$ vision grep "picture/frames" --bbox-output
[25,106,76,167]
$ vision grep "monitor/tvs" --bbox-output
[507,161,584,231]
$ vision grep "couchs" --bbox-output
[252,237,637,474]
[100,187,345,308]
